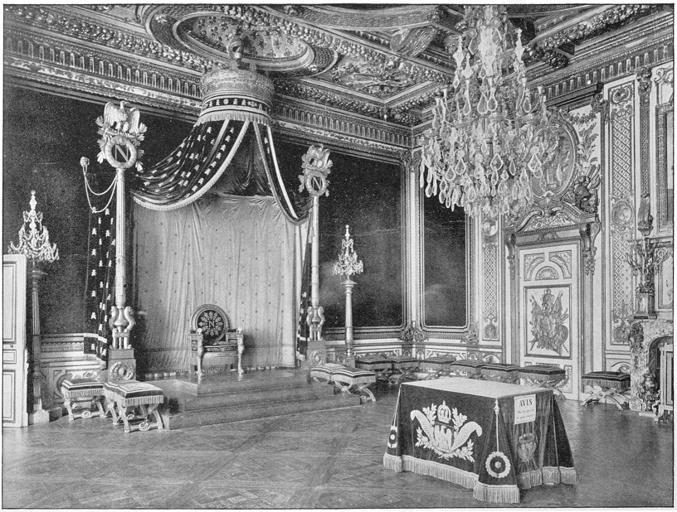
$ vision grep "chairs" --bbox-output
[190,303,245,385]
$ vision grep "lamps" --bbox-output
[9,191,62,425]
[421,4,562,237]
[332,225,363,369]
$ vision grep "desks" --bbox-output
[381,376,577,504]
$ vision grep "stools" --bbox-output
[104,380,164,433]
[312,364,345,383]
[423,358,455,376]
[580,370,630,412]
[480,363,520,386]
[60,378,108,422]
[334,367,376,402]
[450,359,486,379]
[359,358,394,383]
[518,365,566,402]
[391,358,420,384]
